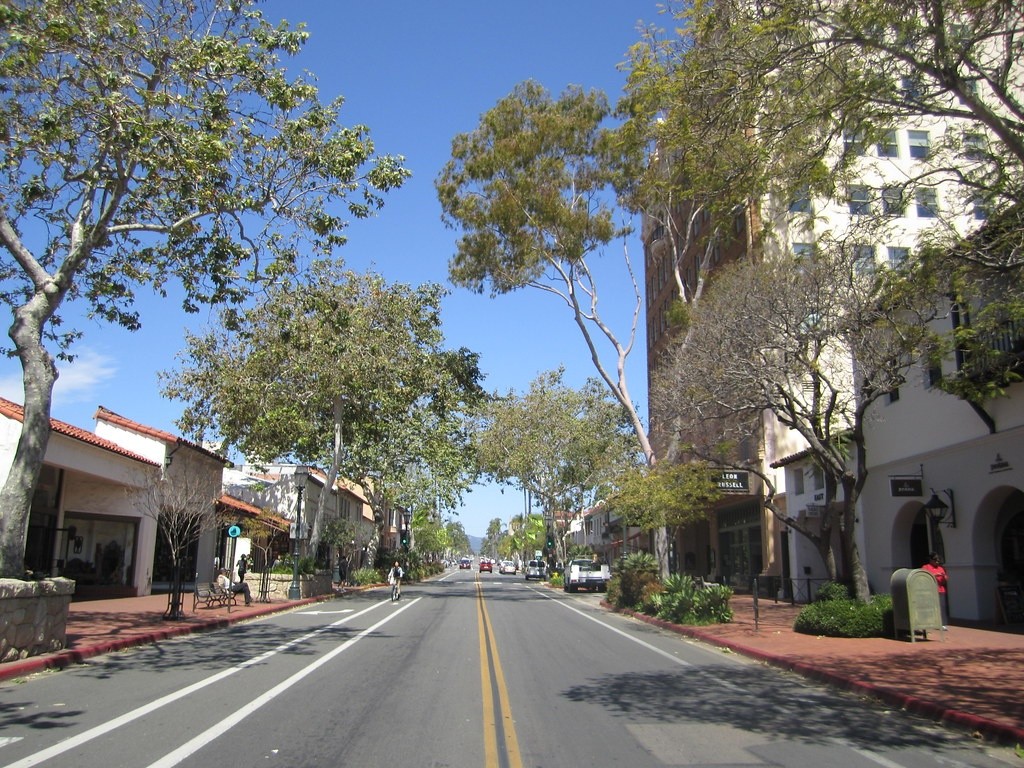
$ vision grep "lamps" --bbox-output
[921,488,956,528]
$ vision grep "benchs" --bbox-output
[193,582,236,612]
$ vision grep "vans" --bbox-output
[524,559,548,582]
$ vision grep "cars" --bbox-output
[478,561,493,573]
[460,559,470,570]
[497,560,517,575]
[440,559,457,568]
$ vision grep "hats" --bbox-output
[219,567,227,571]
[241,554,247,558]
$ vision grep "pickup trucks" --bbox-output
[563,558,611,594]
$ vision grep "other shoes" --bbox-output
[942,626,948,631]
[250,598,254,602]
[245,603,253,607]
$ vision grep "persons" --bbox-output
[336,556,347,594]
[236,554,248,583]
[387,561,402,596]
[922,552,948,631]
[216,568,254,607]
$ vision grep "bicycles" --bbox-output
[389,576,399,602]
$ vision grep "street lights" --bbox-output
[403,508,411,554]
[287,465,310,600]
[543,513,553,572]
